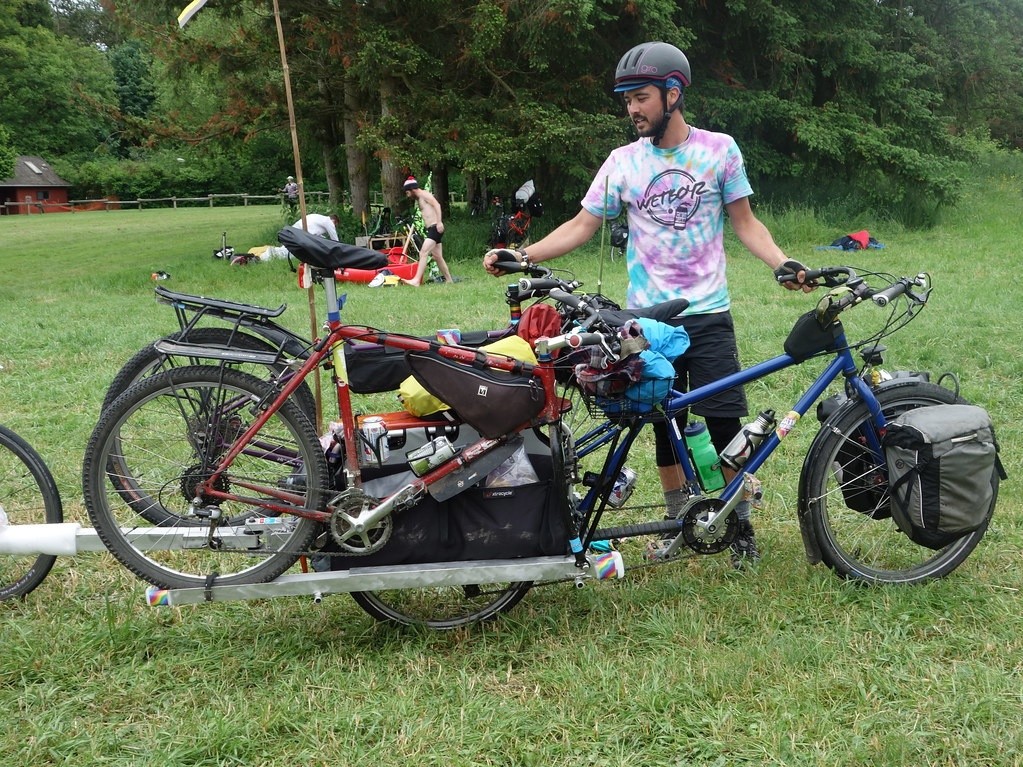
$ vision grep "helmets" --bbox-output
[609,41,693,94]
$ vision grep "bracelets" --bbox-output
[515,247,529,264]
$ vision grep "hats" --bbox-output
[402,176,418,191]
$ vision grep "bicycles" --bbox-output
[0,176,1012,637]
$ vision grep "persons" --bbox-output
[289,213,340,242]
[401,175,456,287]
[483,42,819,571]
[278,176,299,217]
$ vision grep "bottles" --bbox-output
[684,420,726,494]
[862,356,892,386]
[718,410,776,472]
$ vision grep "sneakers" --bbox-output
[642,518,694,562]
[728,518,755,570]
